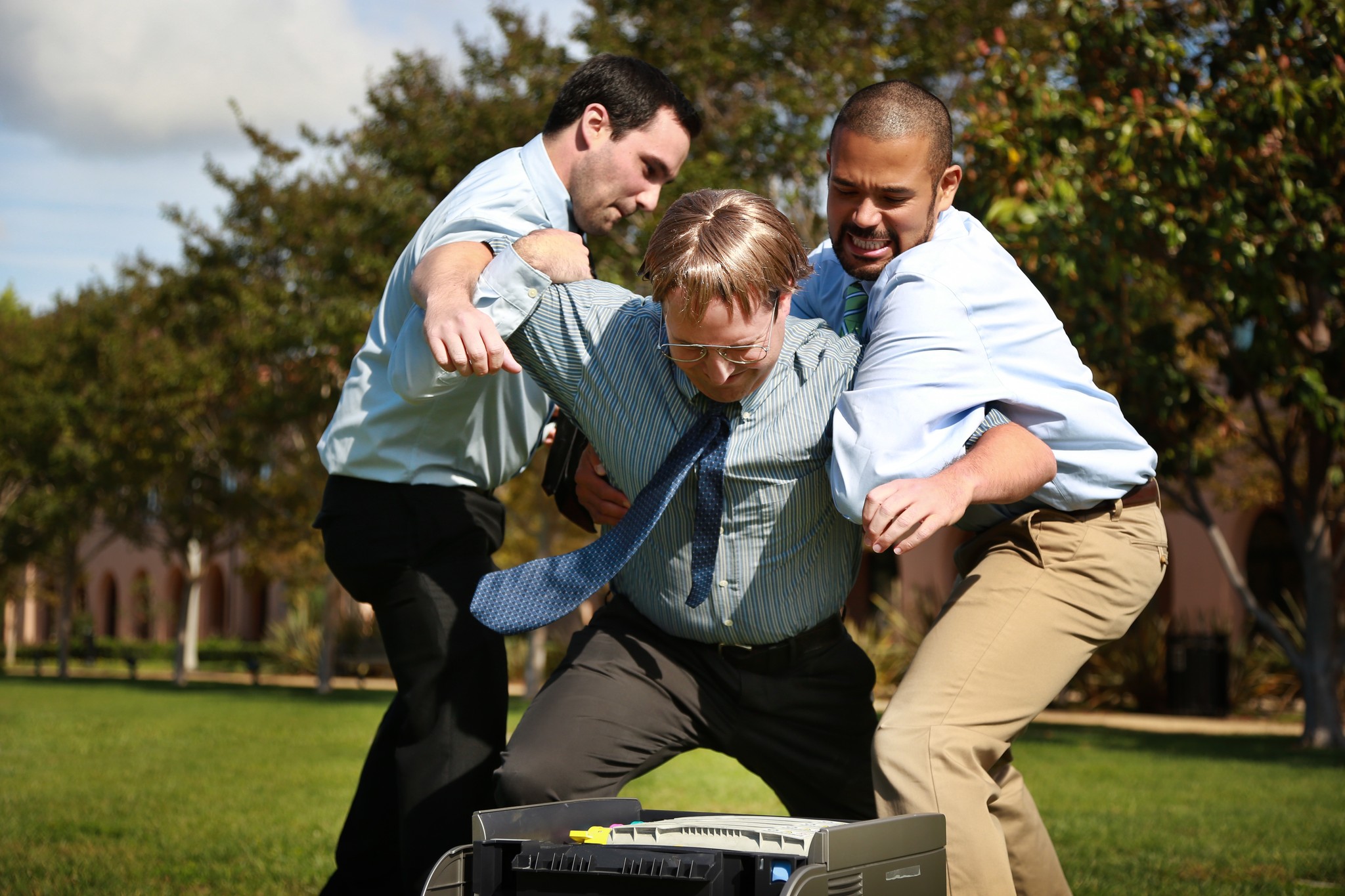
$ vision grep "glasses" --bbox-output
[657,297,777,363]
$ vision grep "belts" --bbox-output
[1068,480,1158,516]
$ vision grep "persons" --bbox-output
[417,185,1060,827]
[303,47,704,896]
[571,79,1174,896]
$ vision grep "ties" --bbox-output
[840,284,867,343]
[470,410,730,634]
